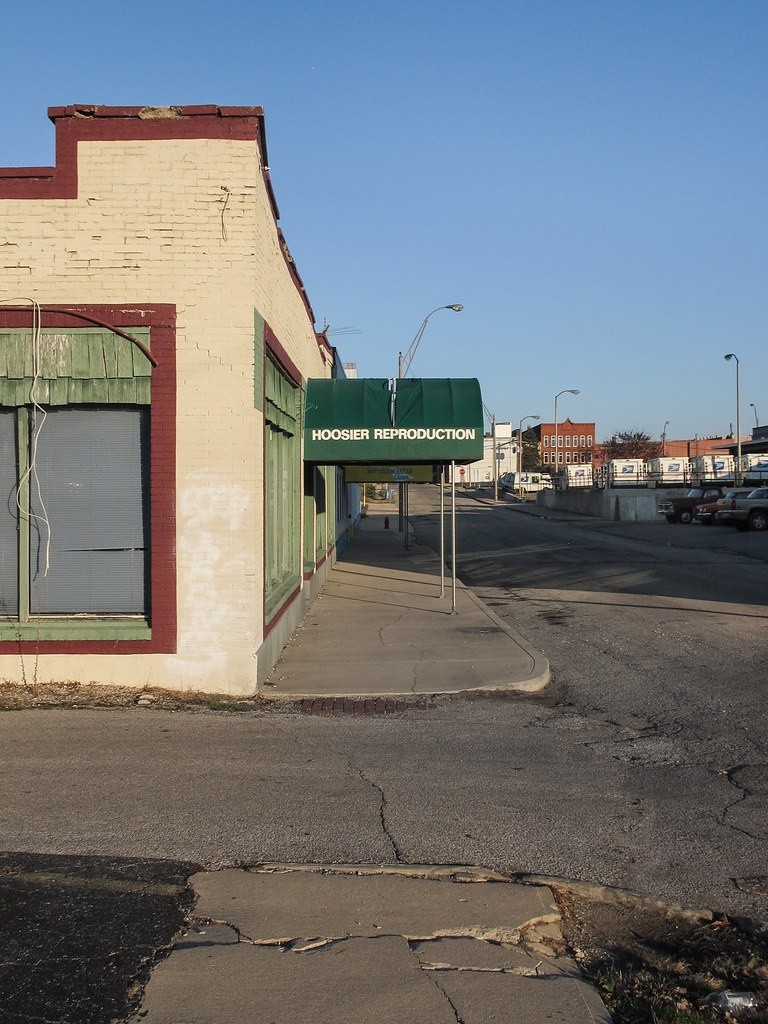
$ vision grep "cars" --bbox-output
[717,487,768,532]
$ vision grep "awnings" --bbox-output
[304,377,484,469]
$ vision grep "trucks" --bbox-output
[503,472,553,492]
[561,464,594,490]
[597,453,768,489]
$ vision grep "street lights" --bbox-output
[397,303,464,534]
[519,415,541,499]
[663,421,672,456]
[554,389,581,482]
[724,354,741,486]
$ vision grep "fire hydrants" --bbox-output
[384,516,390,529]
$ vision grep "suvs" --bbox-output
[690,491,751,524]
[656,486,730,524]
